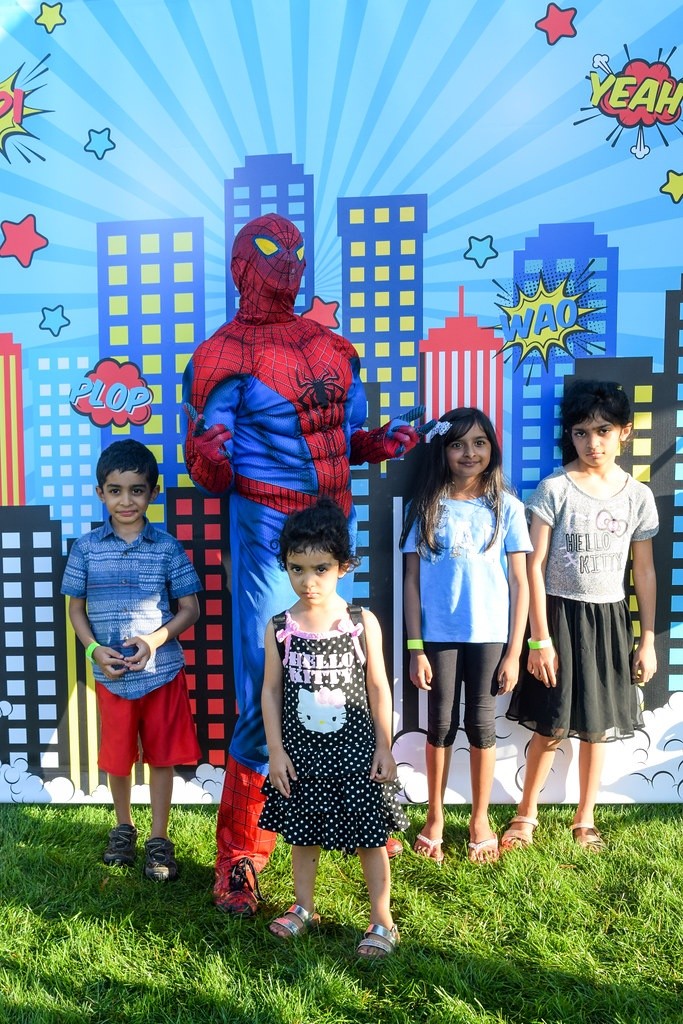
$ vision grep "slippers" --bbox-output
[468,832,500,863]
[414,833,444,862]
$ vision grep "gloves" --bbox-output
[184,402,235,464]
[382,404,437,459]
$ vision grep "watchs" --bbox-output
[86,642,101,666]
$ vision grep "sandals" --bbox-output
[501,816,540,849]
[569,823,606,853]
[265,904,321,941]
[355,923,400,957]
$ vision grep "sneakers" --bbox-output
[103,824,138,867]
[385,839,403,857]
[214,858,269,918]
[143,837,178,882]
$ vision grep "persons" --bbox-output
[397,407,535,864]
[185,213,438,914]
[261,496,401,955]
[59,439,204,880]
[500,384,660,854]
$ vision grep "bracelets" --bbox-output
[407,640,423,650]
[527,637,552,649]
